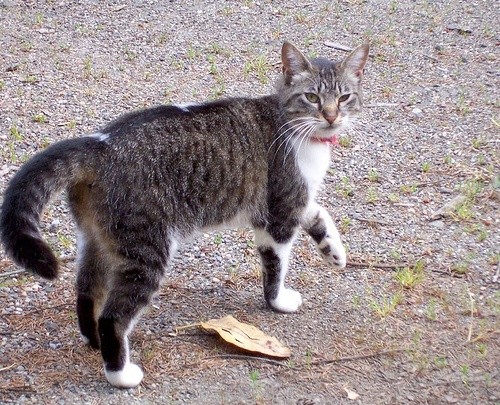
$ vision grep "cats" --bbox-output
[0,40,371,389]
